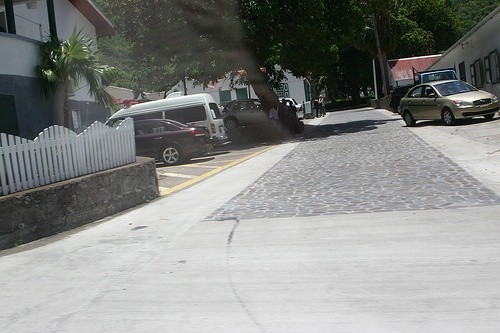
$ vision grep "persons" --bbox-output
[269,99,297,141]
[313,94,326,118]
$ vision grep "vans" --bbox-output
[105,93,232,152]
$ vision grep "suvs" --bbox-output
[389,84,419,115]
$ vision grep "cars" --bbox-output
[134,119,213,166]
[280,98,304,119]
[397,80,500,127]
[222,98,269,130]
